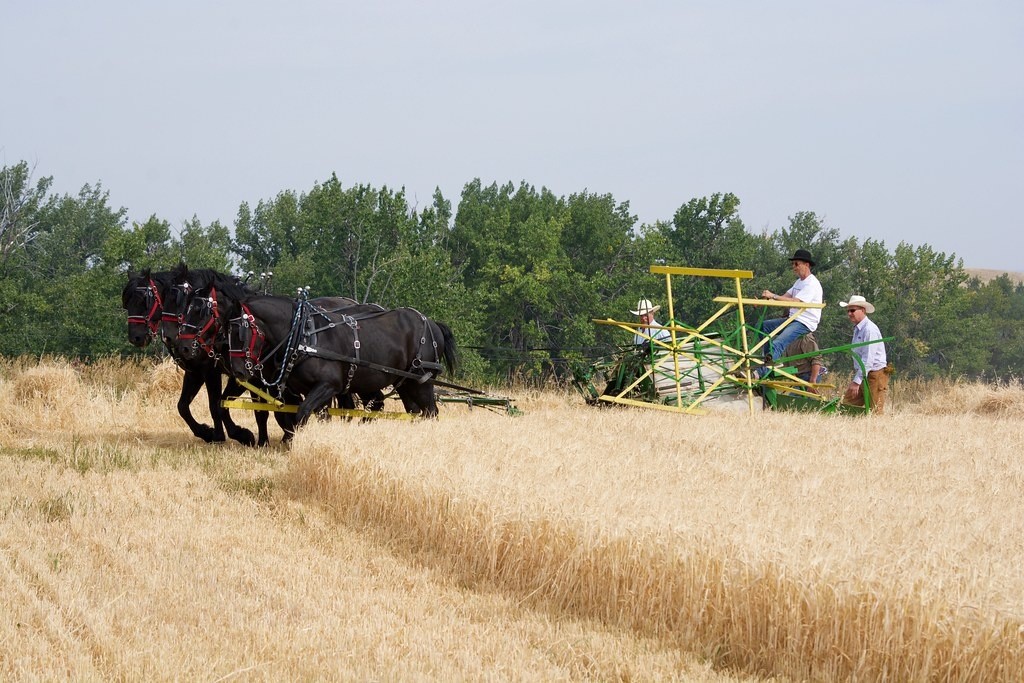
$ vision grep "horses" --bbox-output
[123,260,459,448]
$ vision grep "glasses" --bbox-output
[792,262,798,266]
[847,309,856,312]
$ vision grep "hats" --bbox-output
[630,300,660,316]
[788,250,816,266]
[839,294,875,314]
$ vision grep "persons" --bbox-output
[739,249,823,387]
[838,295,888,416]
[780,334,821,401]
[630,298,672,346]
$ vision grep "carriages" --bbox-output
[121,260,895,448]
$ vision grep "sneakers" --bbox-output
[731,370,758,386]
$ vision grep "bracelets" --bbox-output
[772,294,778,300]
